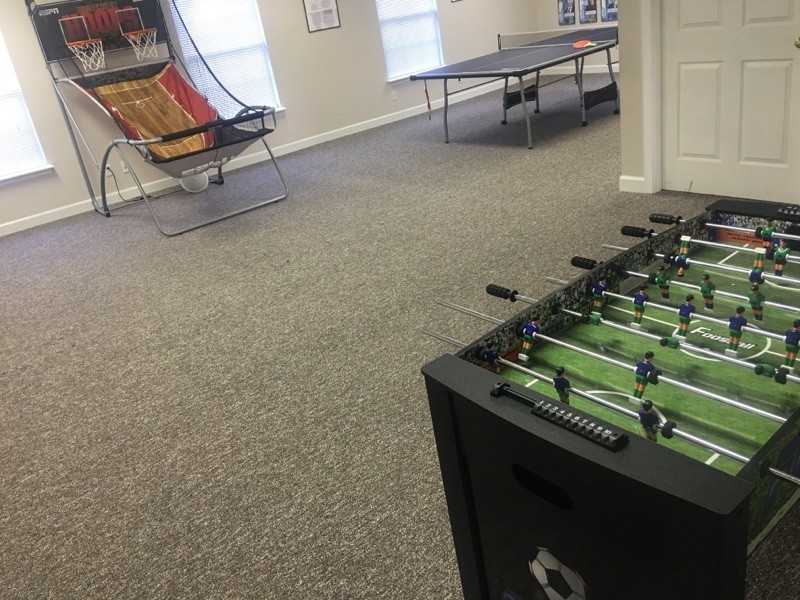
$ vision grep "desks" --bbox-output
[410,26,619,149]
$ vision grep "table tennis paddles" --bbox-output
[572,38,597,49]
